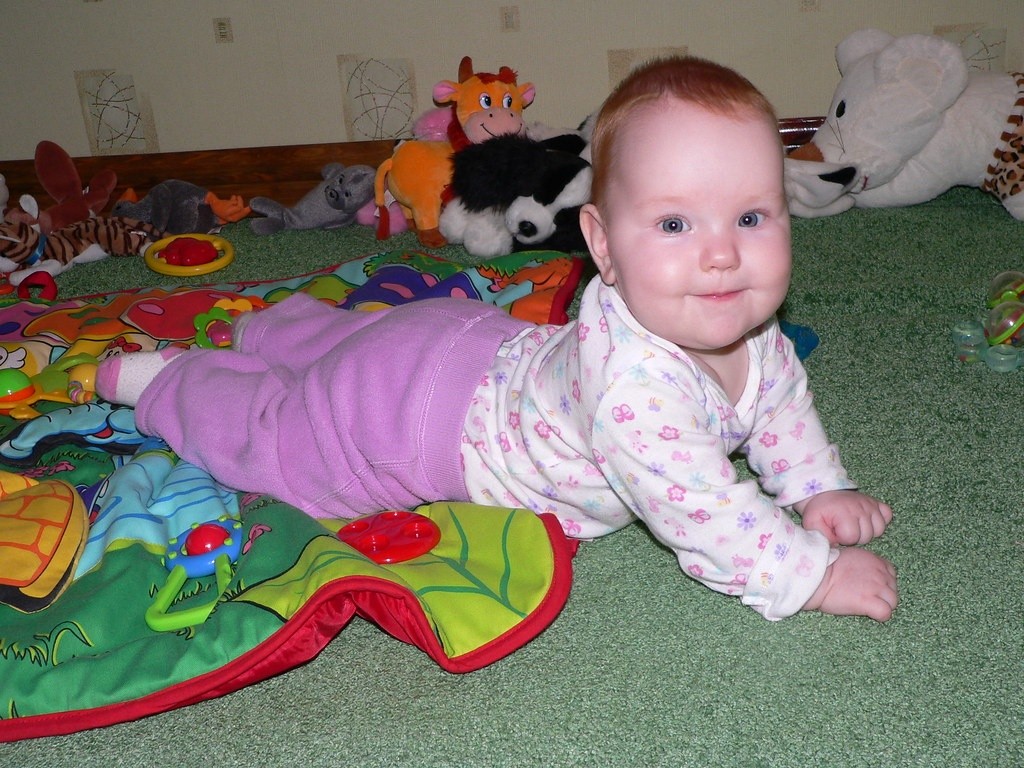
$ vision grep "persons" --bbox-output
[95,55,900,623]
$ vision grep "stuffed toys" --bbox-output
[250,162,378,237]
[356,56,536,248]
[438,133,596,257]
[0,139,251,301]
[787,27,1023,222]
